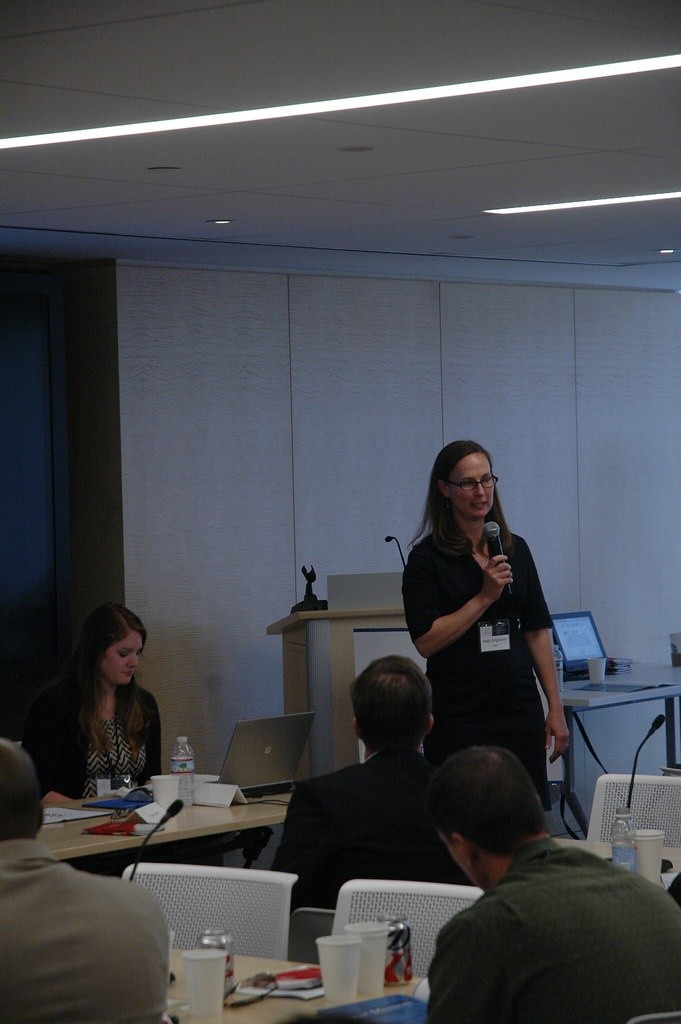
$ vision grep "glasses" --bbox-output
[446,475,498,491]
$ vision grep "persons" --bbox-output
[0,738,171,1024]
[20,600,162,807]
[400,438,572,813]
[268,655,471,910]
[423,744,681,1024]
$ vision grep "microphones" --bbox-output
[627,714,666,808]
[128,799,184,882]
[385,536,405,568]
[483,522,511,596]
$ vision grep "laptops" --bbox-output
[202,710,316,798]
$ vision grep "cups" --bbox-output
[345,922,391,993]
[587,657,607,683]
[315,935,362,1002]
[183,948,229,1015]
[636,829,666,883]
[151,775,179,810]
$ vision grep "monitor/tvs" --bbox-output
[551,610,609,673]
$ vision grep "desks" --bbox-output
[162,950,426,1024]
[552,837,681,891]
[35,792,293,879]
[561,663,681,839]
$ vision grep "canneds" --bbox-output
[376,916,414,986]
[197,929,236,995]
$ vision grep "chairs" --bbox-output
[587,773,681,848]
[332,878,486,979]
[122,862,300,961]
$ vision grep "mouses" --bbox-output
[124,788,154,801]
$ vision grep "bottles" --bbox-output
[609,807,638,874]
[553,645,563,694]
[170,736,195,806]
